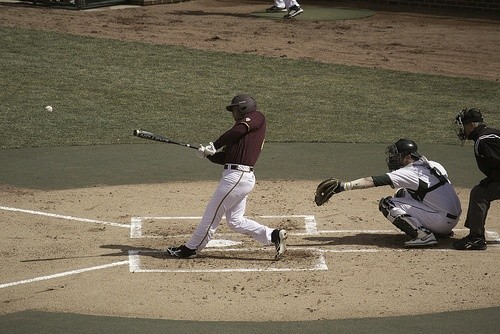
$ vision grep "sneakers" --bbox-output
[404,231,437,247]
[283,6,303,19]
[266,5,287,12]
[273,229,288,260]
[454,234,487,250]
[167,245,197,259]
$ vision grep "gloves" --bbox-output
[198,142,216,158]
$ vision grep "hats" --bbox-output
[462,108,484,123]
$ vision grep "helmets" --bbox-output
[396,138,421,158]
[225,95,256,116]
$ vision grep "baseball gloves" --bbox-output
[315,177,342,207]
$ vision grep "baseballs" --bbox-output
[45,105,52,112]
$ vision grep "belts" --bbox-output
[224,165,253,172]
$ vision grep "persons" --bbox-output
[453,108,500,250]
[315,138,462,246]
[266,0,304,19]
[167,95,287,261]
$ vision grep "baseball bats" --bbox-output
[132,129,199,150]
